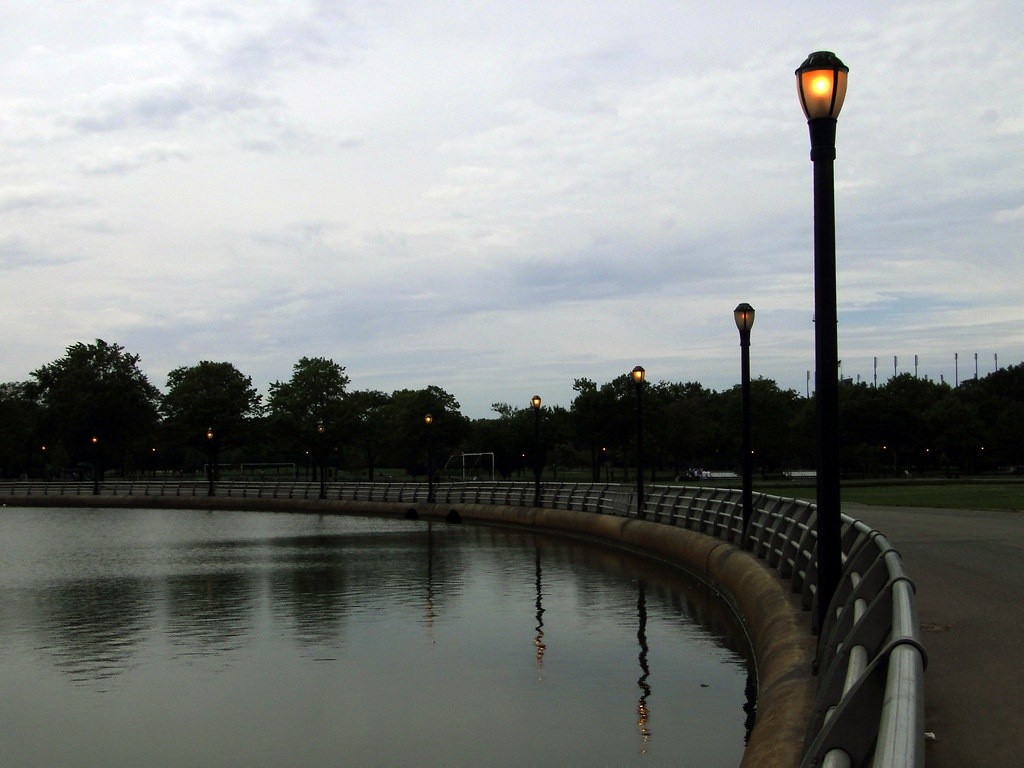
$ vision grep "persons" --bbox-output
[690,467,704,480]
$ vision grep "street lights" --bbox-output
[733,303,755,552]
[205,427,216,497]
[317,418,328,500]
[425,413,435,503]
[793,50,849,658]
[632,365,646,520]
[531,395,541,508]
[91,434,99,495]
